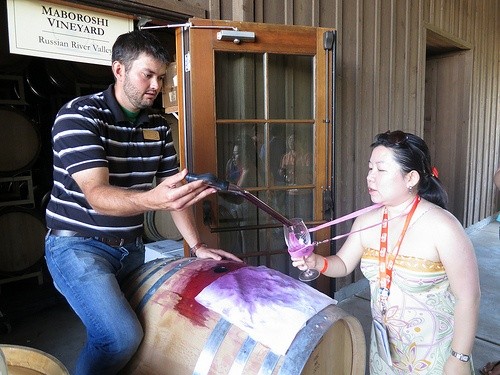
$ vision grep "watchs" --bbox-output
[452,351,471,362]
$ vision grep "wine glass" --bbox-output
[283,218,320,281]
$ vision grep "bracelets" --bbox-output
[191,242,207,257]
[320,258,328,274]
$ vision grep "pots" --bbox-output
[0,344,70,375]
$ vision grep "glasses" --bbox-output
[384,130,418,164]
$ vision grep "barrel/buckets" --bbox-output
[120,257,366,375]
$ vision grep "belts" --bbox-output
[46,225,139,247]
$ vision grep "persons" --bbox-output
[224,125,312,265]
[290,130,481,375]
[43,31,244,375]
